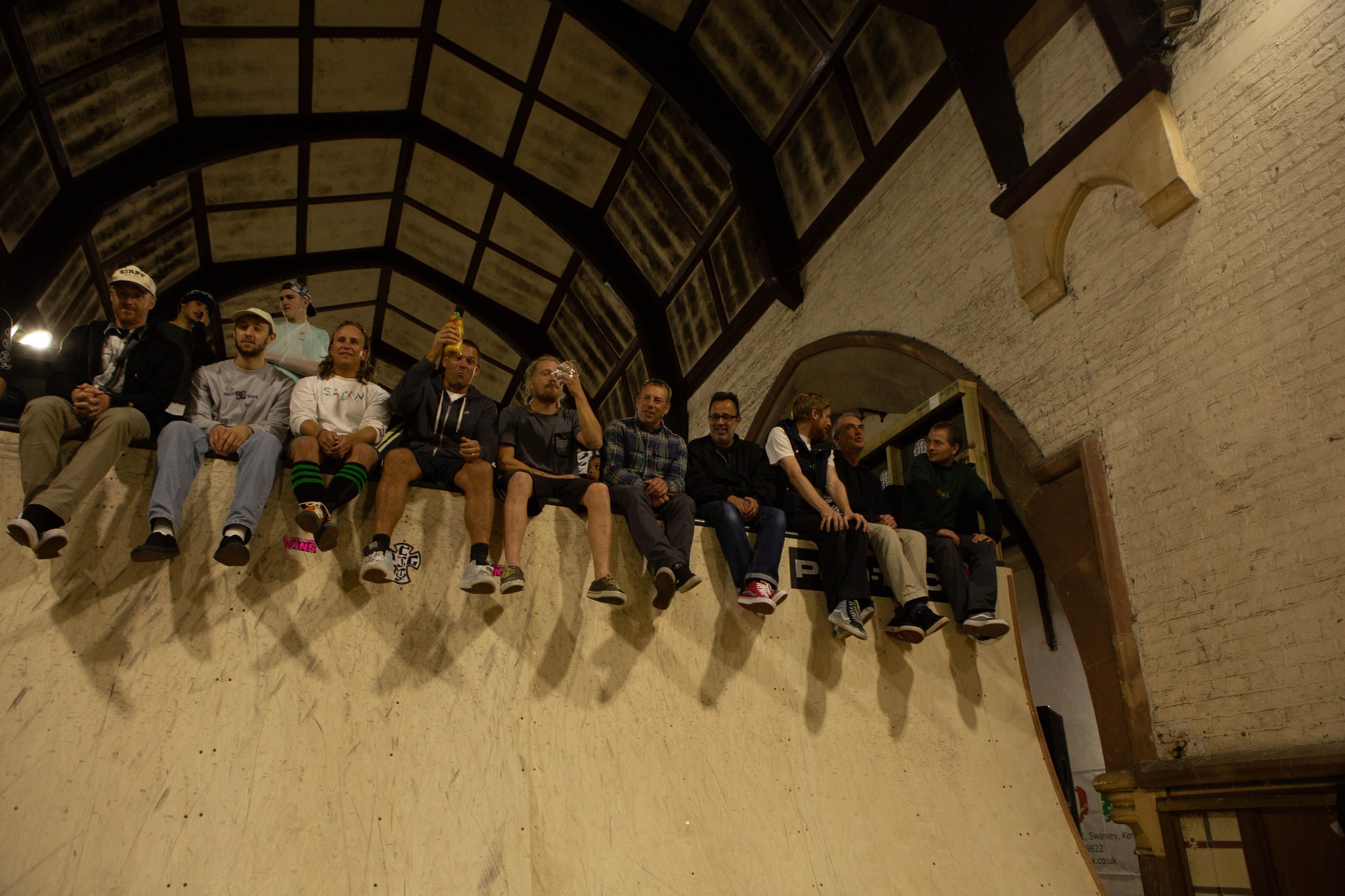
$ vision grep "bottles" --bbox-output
[444,311,464,359]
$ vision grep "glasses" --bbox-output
[708,415,739,421]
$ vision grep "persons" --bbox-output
[828,409,948,644]
[287,320,391,542]
[765,392,876,639]
[140,288,223,449]
[601,378,703,610]
[5,264,191,561]
[495,355,627,605]
[683,391,787,614]
[131,306,296,566]
[362,316,500,596]
[908,419,1010,647]
[585,454,601,482]
[258,279,331,381]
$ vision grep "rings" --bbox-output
[471,452,476,459]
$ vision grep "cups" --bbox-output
[550,359,582,379]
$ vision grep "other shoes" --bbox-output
[5,509,40,549]
[213,535,250,567]
[676,563,702,594]
[652,566,677,610]
[32,514,68,559]
[130,532,180,563]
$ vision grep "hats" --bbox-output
[230,307,274,333]
[280,282,317,317]
[178,290,214,316]
[109,265,157,299]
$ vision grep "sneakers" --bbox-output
[885,601,950,644]
[313,517,340,552]
[459,554,497,594]
[499,561,526,595]
[828,598,875,640]
[294,501,331,533]
[963,611,1011,645]
[586,574,627,605]
[738,580,788,617]
[360,541,395,584]
